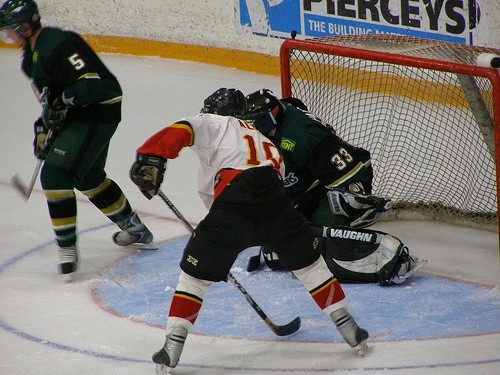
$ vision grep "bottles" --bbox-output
[476,52,500,68]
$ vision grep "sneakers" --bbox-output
[329,306,371,357]
[56,235,78,282]
[379,235,428,285]
[151,326,188,375]
[111,210,159,252]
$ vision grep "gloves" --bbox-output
[33,115,57,159]
[128,152,168,200]
[41,94,70,132]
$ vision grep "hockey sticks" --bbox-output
[155,186,302,337]
[246,245,263,272]
[9,129,53,203]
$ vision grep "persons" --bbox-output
[246,88,427,287]
[129,88,370,375]
[0,0,159,282]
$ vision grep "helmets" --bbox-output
[245,89,288,133]
[204,87,248,118]
[0,0,41,35]
